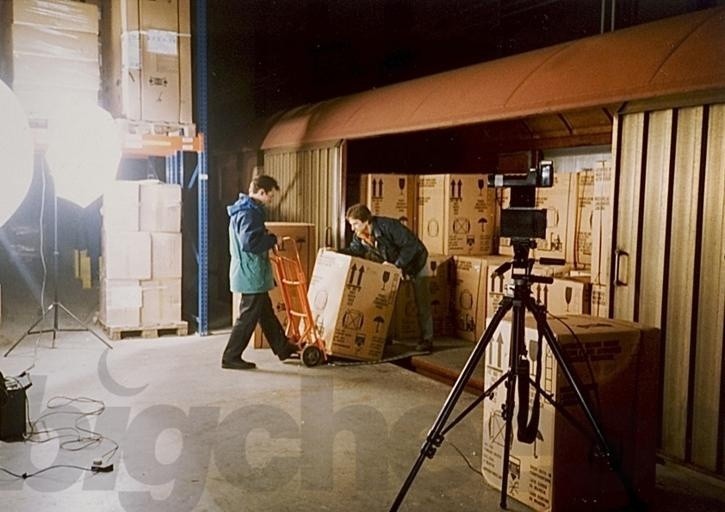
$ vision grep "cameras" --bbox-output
[487,160,554,241]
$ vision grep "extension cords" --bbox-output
[91,464,115,473]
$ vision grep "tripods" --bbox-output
[5,172,113,357]
[389,240,643,512]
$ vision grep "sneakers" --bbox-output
[415,341,433,352]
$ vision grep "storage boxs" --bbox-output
[1,0,99,34]
[142,183,183,229]
[98,277,142,327]
[358,172,414,224]
[449,251,511,340]
[101,180,142,230]
[571,171,594,268]
[590,287,605,319]
[487,267,548,317]
[594,160,609,287]
[298,248,401,360]
[1,61,100,88]
[548,278,589,313]
[498,169,566,260]
[151,232,185,276]
[104,231,153,280]
[1,25,104,59]
[396,256,451,340]
[417,172,496,258]
[481,314,664,512]
[141,278,184,323]
[104,0,194,126]
[233,221,316,349]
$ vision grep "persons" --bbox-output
[326,204,435,353]
[220,175,306,370]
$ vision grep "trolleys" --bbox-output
[268,236,327,368]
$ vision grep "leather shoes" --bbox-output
[222,359,255,370]
[278,342,307,360]
